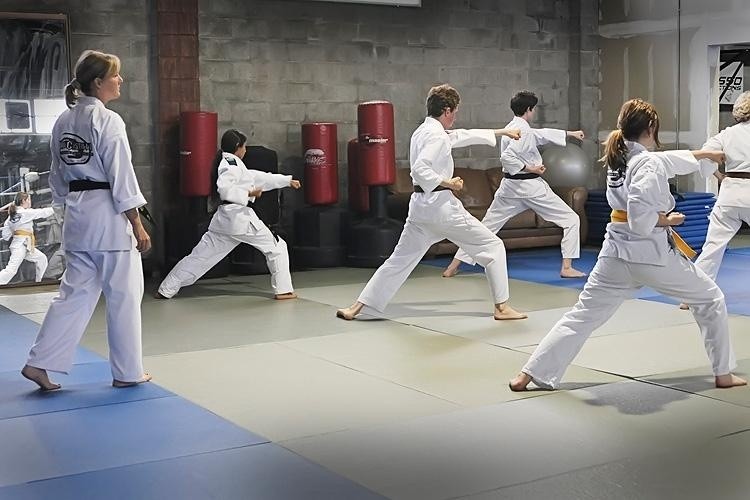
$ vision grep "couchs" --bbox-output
[387,165,588,252]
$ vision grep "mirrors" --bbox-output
[0,11,81,289]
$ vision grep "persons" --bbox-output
[510,100,747,391]
[680,91,750,310]
[154,128,300,300]
[443,90,585,277]
[336,84,528,320]
[20,50,152,390]
[0,191,56,286]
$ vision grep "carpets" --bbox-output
[0,303,388,500]
[427,250,749,320]
